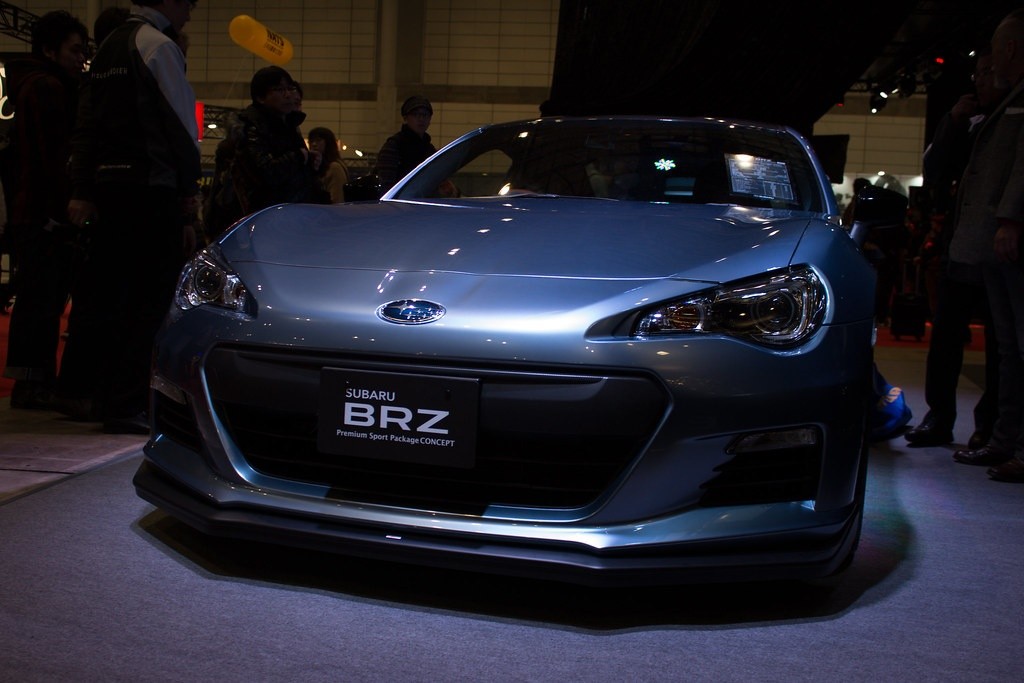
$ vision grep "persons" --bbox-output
[0,0,351,437]
[953,11,1024,482]
[844,179,946,328]
[372,95,438,199]
[904,48,1000,450]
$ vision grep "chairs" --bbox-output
[647,154,724,205]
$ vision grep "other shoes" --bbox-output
[905,411,954,445]
[53,398,94,419]
[953,433,987,459]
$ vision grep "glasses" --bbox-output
[408,112,429,118]
[272,87,296,95]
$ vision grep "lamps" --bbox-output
[870,75,917,115]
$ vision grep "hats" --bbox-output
[401,95,433,116]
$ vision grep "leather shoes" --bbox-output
[958,444,1014,466]
[103,410,150,434]
[986,456,1024,483]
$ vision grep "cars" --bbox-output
[132,112,895,593]
